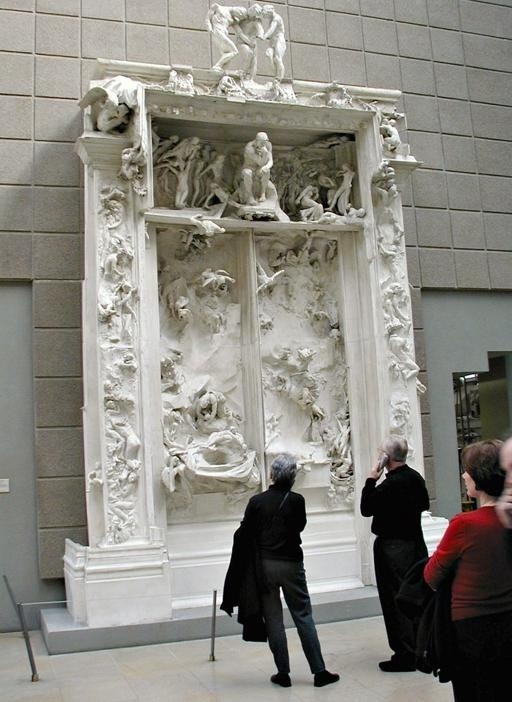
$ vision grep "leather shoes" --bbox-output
[271,671,291,686]
[379,661,416,672]
[314,670,340,687]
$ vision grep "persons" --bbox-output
[496,436,512,572]
[241,456,339,687]
[359,434,430,672]
[424,439,512,702]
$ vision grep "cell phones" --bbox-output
[377,452,388,472]
